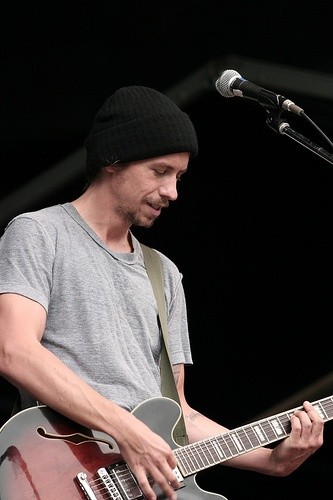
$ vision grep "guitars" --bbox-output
[0,394,333,500]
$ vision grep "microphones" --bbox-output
[215,69,305,116]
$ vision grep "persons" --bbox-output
[0,85,333,500]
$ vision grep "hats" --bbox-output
[85,86,198,169]
